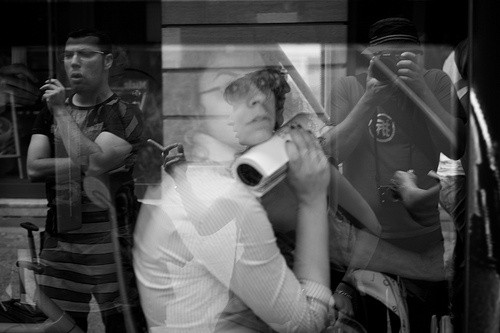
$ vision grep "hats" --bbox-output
[359,17,423,57]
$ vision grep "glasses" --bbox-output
[58,49,105,65]
[197,69,270,106]
[270,68,290,90]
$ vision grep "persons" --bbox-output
[131,13,469,333]
[28,25,151,333]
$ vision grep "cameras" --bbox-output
[371,53,409,82]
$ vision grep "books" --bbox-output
[230,113,336,198]
